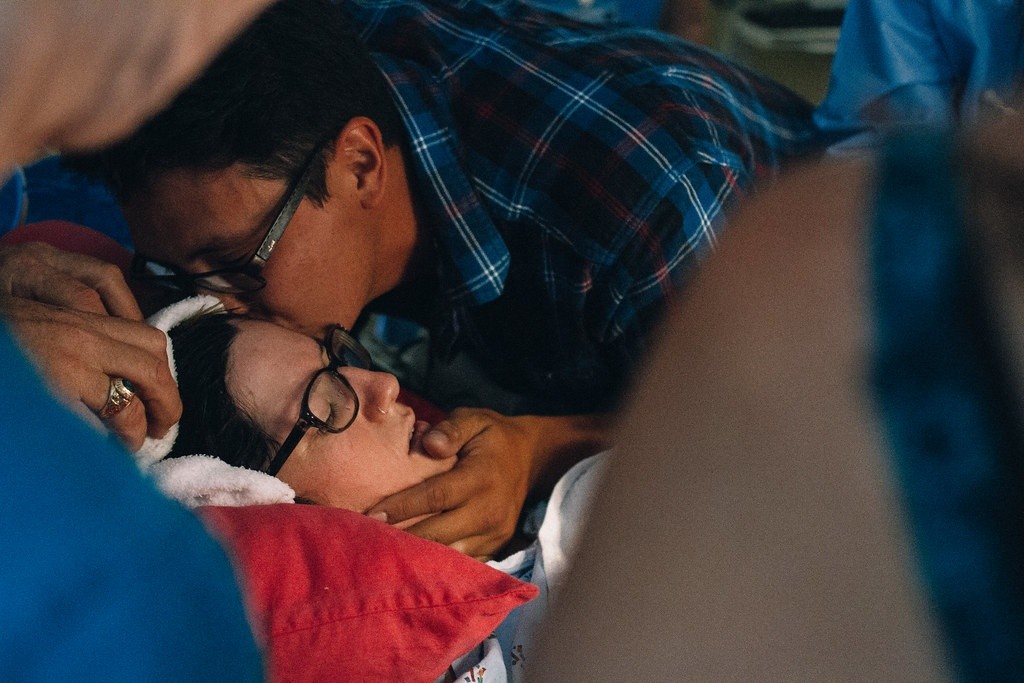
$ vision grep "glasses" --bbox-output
[145,143,325,308]
[267,323,373,484]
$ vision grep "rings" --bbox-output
[99,377,137,420]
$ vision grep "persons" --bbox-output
[0,0,1024,683]
